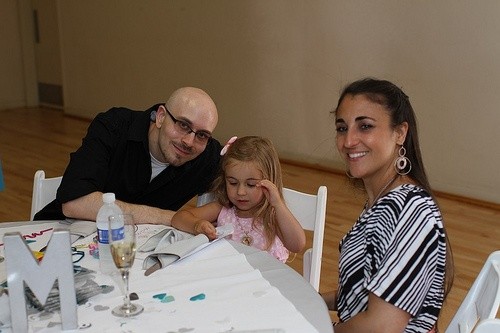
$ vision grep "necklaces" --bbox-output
[369,177,395,207]
[233,207,254,246]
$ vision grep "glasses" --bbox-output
[163,105,213,147]
[71,246,85,264]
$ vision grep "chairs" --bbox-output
[445,249,500,333]
[218,185,328,292]
[30,170,214,222]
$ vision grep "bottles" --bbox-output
[96,192,124,275]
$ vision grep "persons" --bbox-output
[34,87,223,224]
[318,79,454,333]
[171,136,306,263]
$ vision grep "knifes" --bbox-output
[144,261,161,276]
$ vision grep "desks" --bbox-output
[0,221,335,333]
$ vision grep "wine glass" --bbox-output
[108,214,144,317]
